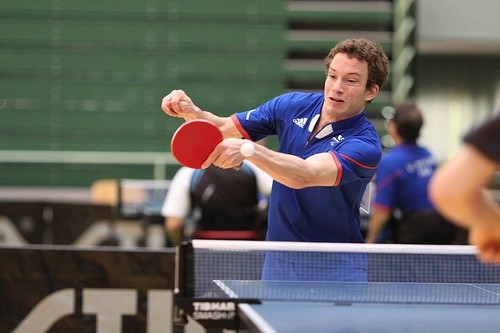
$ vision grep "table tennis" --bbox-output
[240,140,257,157]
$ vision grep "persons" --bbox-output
[162,38,389,282]
[163,159,274,249]
[365,100,500,283]
